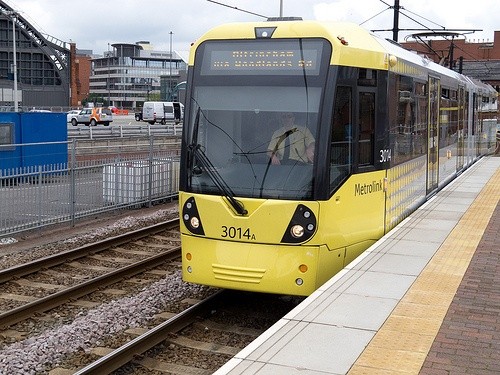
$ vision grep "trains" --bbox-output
[179,21,498,300]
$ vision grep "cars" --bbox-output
[135,110,144,121]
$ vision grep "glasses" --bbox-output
[282,114,293,119]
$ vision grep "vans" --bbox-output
[142,101,185,124]
[66,110,83,122]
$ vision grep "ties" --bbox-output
[283,135,290,161]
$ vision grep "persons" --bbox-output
[266,112,315,166]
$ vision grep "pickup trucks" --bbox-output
[71,107,113,126]
